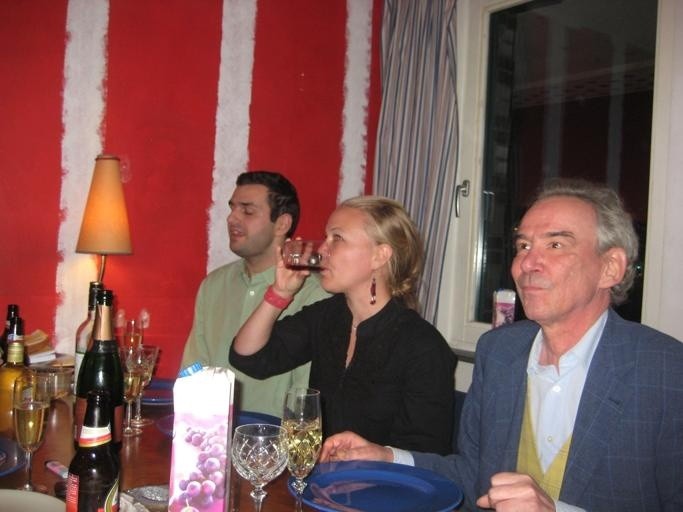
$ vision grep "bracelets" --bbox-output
[264,285,294,309]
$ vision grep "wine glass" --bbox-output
[230,387,323,512]
[12,375,54,491]
[121,320,159,436]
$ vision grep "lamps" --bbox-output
[75,154,132,283]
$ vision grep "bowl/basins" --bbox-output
[30,365,75,399]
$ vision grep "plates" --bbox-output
[0,488,66,512]
[0,438,33,476]
[160,409,281,439]
[285,459,465,511]
[142,379,174,406]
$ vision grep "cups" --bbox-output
[284,240,328,271]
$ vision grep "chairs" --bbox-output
[448,349,476,452]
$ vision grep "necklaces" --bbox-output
[351,322,358,332]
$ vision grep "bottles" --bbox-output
[73,290,123,451]
[73,281,104,407]
[0,318,35,440]
[0,304,19,367]
[65,390,120,512]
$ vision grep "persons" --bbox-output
[228,195,458,457]
[179,170,336,420]
[319,178,683,512]
